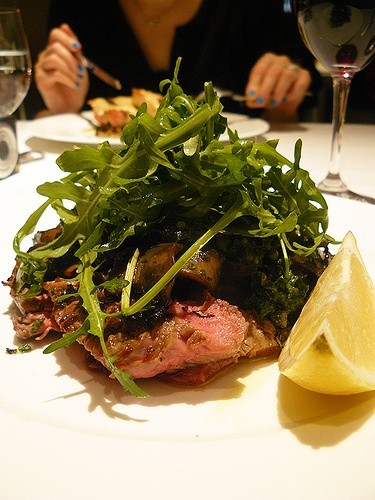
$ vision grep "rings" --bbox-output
[287,66,298,72]
[41,57,49,70]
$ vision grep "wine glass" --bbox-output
[296,0,375,199]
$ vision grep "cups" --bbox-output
[0,7,32,119]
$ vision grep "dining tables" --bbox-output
[1,116,375,499]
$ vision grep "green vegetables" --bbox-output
[14,58,343,397]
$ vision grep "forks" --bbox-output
[196,86,313,101]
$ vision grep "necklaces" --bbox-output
[134,0,181,26]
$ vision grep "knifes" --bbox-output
[77,53,122,91]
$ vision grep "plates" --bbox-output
[0,191,375,439]
[29,110,271,151]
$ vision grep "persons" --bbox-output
[14,0,323,123]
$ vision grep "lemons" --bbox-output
[278,230,375,396]
